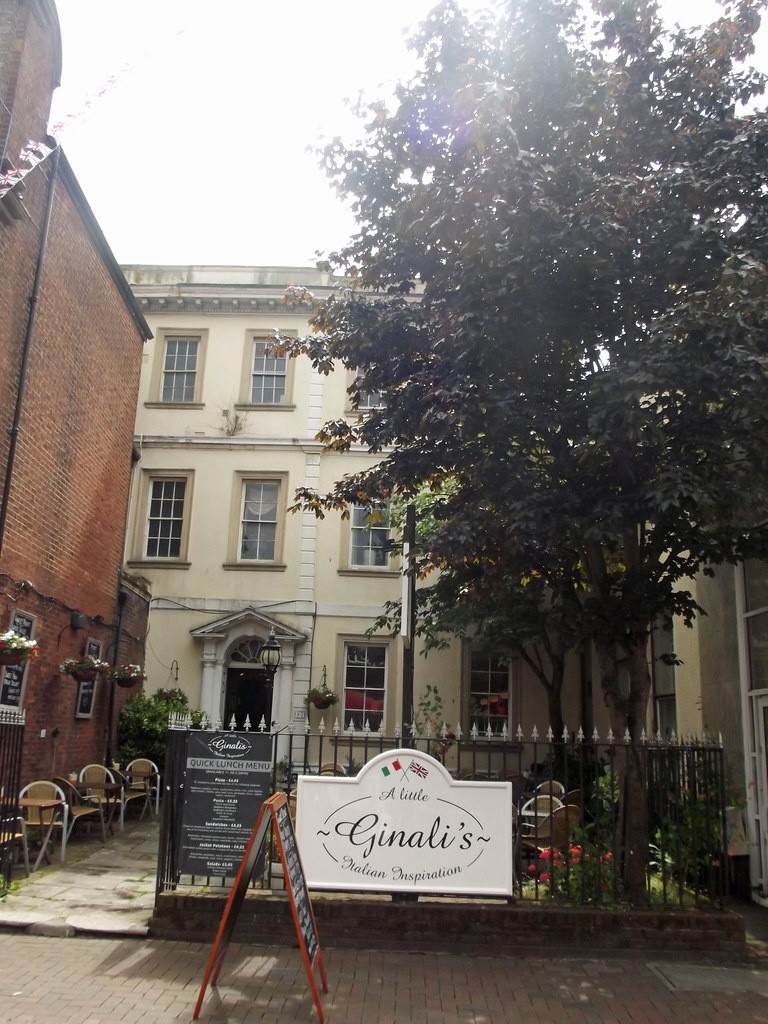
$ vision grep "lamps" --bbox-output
[94,615,104,622]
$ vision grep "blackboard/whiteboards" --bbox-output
[206,792,322,978]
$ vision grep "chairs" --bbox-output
[0,759,159,882]
[462,771,581,868]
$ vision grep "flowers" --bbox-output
[152,688,188,706]
[106,664,147,681]
[58,656,110,673]
[303,687,339,705]
[0,629,39,659]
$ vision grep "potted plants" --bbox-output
[264,831,285,889]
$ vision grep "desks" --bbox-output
[123,771,156,820]
[74,783,125,835]
[517,810,550,865]
[3,799,63,864]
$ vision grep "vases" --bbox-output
[0,648,23,665]
[314,697,330,708]
[117,678,137,688]
[72,669,96,681]
